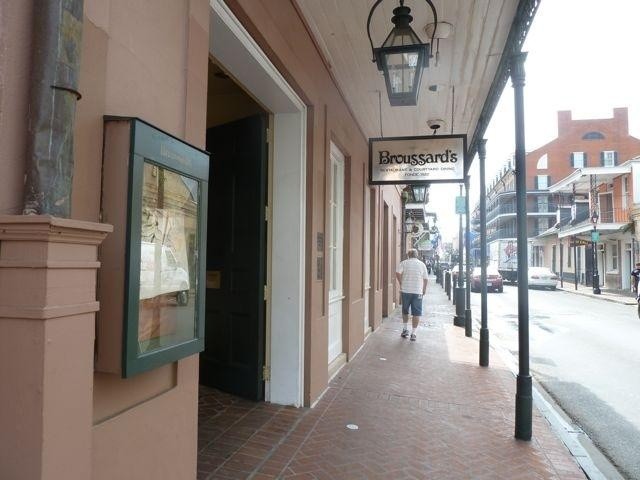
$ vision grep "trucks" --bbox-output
[490,239,535,282]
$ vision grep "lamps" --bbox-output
[407,183,430,203]
[365,0,438,107]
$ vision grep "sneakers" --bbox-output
[401,328,409,337]
[410,334,416,341]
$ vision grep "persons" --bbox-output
[425,257,435,274]
[630,263,640,299]
[396,248,428,341]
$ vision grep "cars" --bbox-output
[140,242,190,304]
[528,267,558,291]
[440,261,503,293]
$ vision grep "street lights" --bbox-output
[590,210,601,294]
